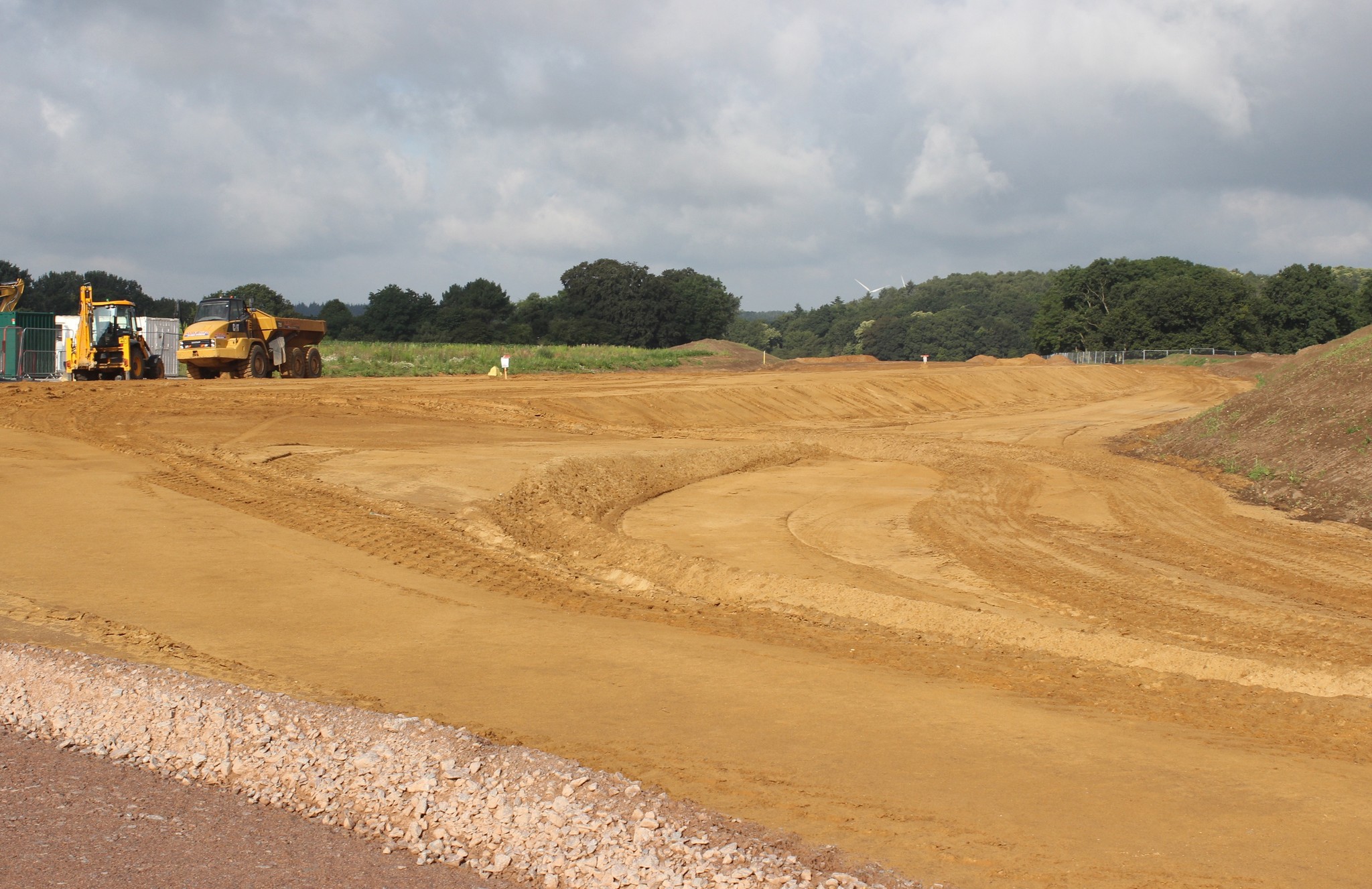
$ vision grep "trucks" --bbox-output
[176,294,328,378]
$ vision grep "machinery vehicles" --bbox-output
[0,277,25,313]
[65,280,166,382]
